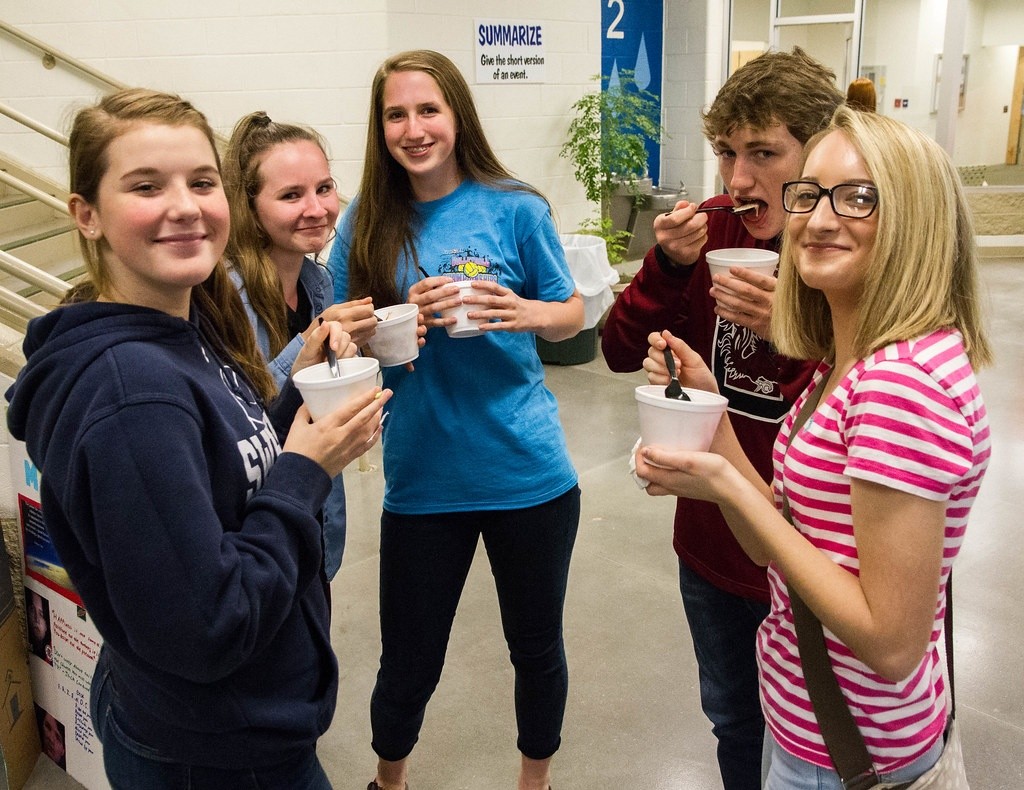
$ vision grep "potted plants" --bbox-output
[558,68,674,335]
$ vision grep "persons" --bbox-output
[325,49,584,790]
[5,88,427,790]
[42,713,65,772]
[28,590,52,663]
[600,48,992,790]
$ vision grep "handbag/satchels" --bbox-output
[869,714,970,790]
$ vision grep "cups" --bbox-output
[705,247,780,313]
[292,357,380,423]
[358,303,420,368]
[634,385,729,471]
[436,280,493,338]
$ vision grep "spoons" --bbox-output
[665,201,762,216]
[663,346,691,402]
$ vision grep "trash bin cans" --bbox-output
[535,233,606,366]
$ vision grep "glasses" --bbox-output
[781,180,877,219]
